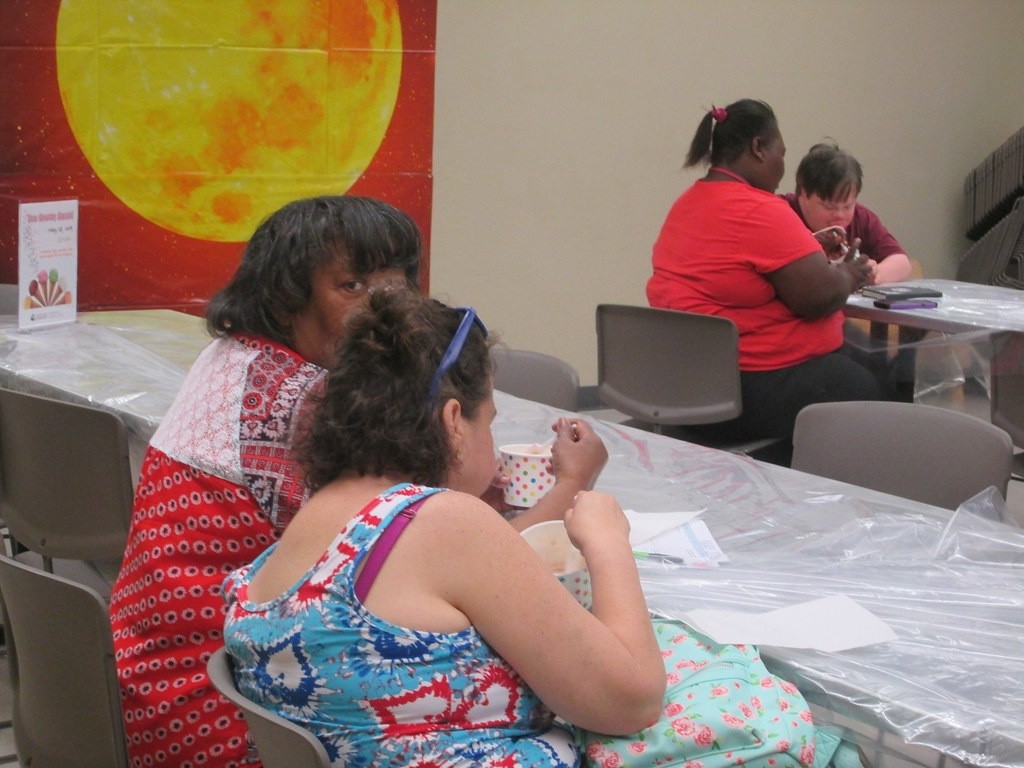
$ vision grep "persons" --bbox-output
[646,99,893,435]
[775,143,911,285]
[111,195,609,768]
[220,280,666,768]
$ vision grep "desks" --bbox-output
[0,306,1024,768]
[843,278,1024,403]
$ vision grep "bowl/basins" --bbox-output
[521,520,592,610]
[498,444,556,507]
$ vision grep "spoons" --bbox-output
[565,541,581,573]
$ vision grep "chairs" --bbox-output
[596,303,790,466]
[790,400,1014,522]
[988,330,1024,481]
[208,646,330,768]
[1,533,129,768]
[0,385,135,575]
[487,348,579,412]
